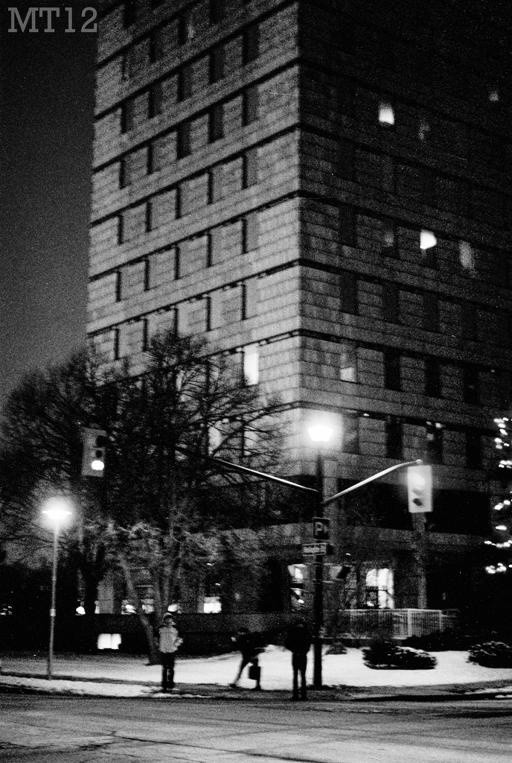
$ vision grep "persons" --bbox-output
[282,616,312,701]
[152,612,185,693]
[229,627,265,691]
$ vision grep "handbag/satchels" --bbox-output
[248,666,260,680]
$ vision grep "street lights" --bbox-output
[45,499,72,679]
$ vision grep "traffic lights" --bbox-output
[81,427,109,477]
[406,464,432,514]
